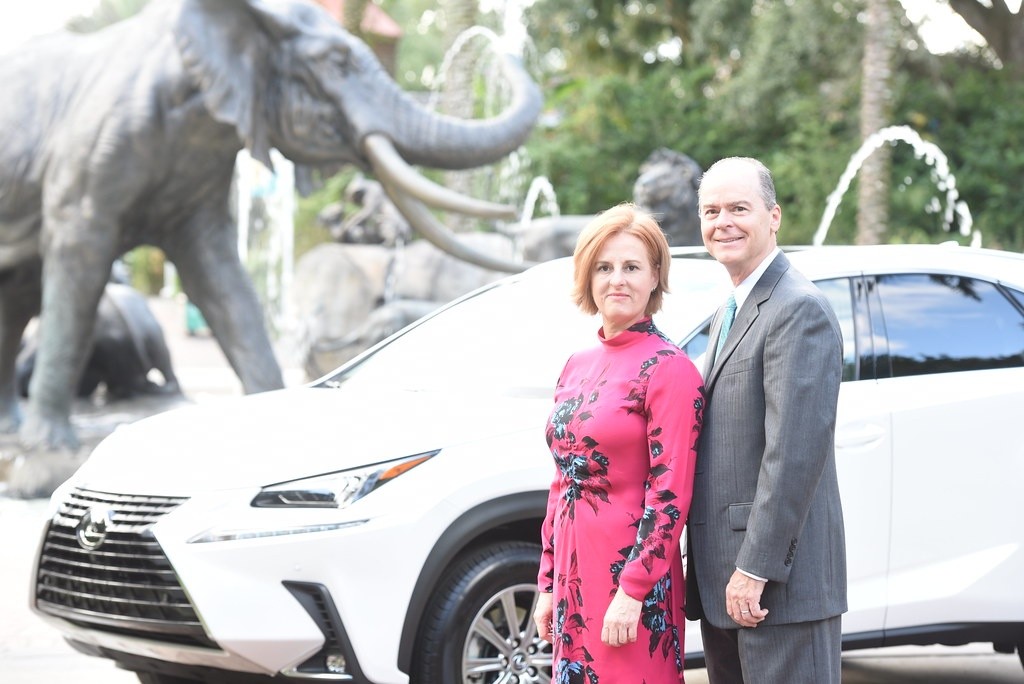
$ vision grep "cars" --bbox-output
[30,238,1024,684]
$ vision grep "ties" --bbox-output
[714,295,738,366]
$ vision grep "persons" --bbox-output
[531,203,706,684]
[682,155,849,684]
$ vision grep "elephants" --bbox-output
[0,0,542,502]
